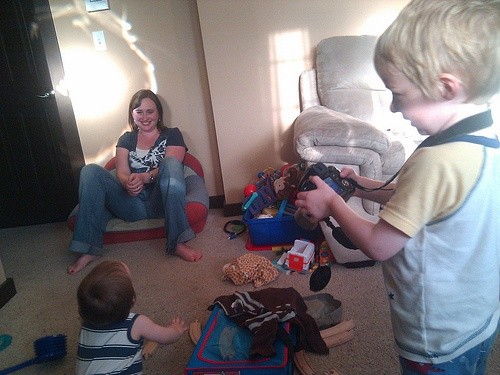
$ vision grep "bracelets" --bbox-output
[149,170,154,184]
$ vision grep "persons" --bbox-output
[74,258,187,375]
[294,0,500,375]
[67,90,203,274]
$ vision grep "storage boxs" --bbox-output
[243,209,306,245]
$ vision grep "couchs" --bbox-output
[293,35,431,268]
[67,154,209,243]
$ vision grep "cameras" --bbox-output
[294,161,355,230]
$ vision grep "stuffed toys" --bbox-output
[273,165,299,204]
